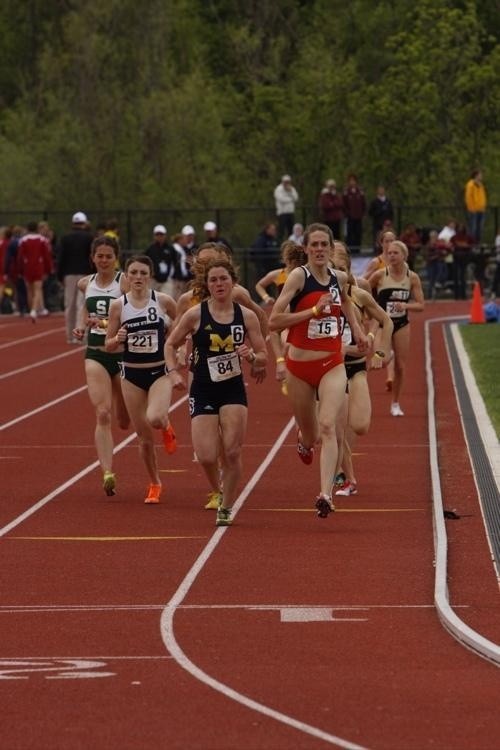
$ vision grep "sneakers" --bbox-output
[296,426,314,465]
[334,472,346,488]
[214,505,234,527]
[102,469,117,497]
[161,421,178,455]
[390,401,404,419]
[65,337,83,348]
[335,476,359,497]
[143,481,163,505]
[12,308,50,324]
[203,489,223,510]
[385,378,395,393]
[314,493,335,519]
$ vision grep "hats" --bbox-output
[151,224,167,236]
[180,224,195,235]
[203,221,217,233]
[70,211,87,226]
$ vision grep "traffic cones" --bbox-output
[468,280,489,325]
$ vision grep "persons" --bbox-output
[104,217,120,243]
[364,230,424,416]
[464,171,487,243]
[146,224,175,298]
[378,223,499,299]
[106,255,178,504]
[342,174,366,252]
[54,211,97,344]
[256,223,393,517]
[367,186,393,253]
[165,222,267,524]
[318,179,344,240]
[73,235,131,496]
[274,174,300,242]
[0,221,56,323]
